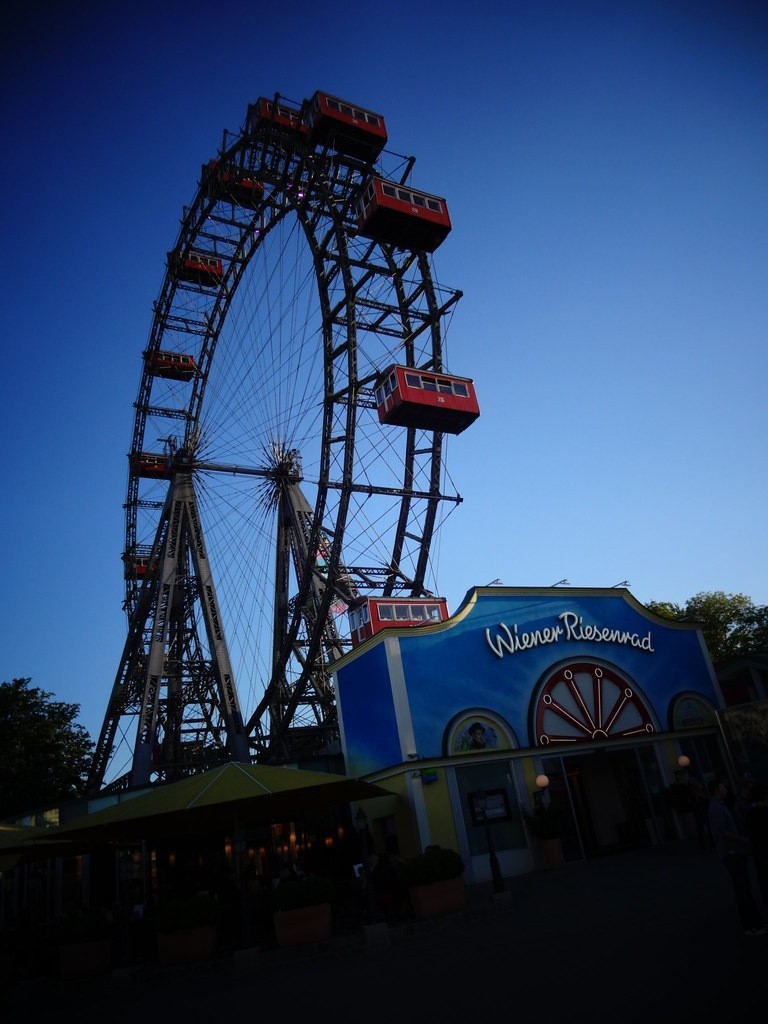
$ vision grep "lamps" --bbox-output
[674,756,690,782]
[534,773,550,801]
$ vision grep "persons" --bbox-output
[704,775,768,936]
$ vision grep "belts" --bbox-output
[727,850,736,854]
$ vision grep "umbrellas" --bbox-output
[25,756,399,888]
[0,822,71,850]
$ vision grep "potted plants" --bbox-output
[271,875,333,947]
[51,911,114,977]
[660,779,698,830]
[522,794,572,872]
[399,841,465,920]
[153,896,217,967]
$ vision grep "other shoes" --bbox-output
[744,927,766,936]
[759,924,768,933]
